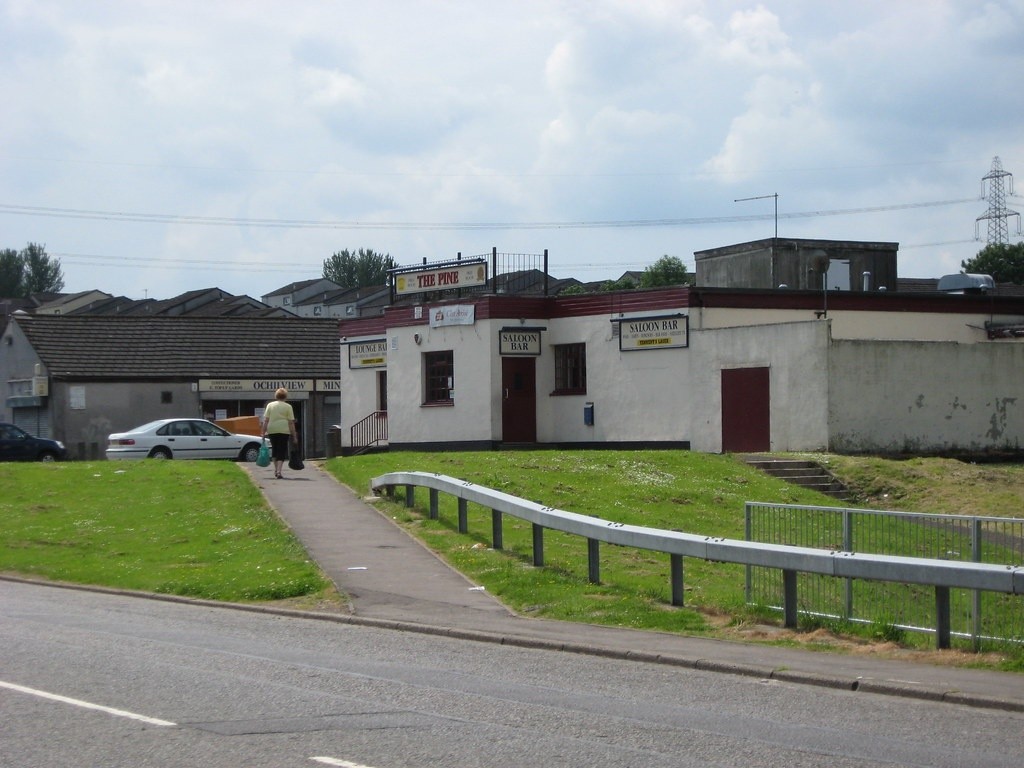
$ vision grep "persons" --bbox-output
[261,387,298,479]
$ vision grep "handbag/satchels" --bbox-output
[256,441,271,467]
[288,444,304,470]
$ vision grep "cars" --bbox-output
[104,417,273,462]
[0,422,67,464]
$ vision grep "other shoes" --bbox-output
[275,471,277,477]
[276,472,282,479]
